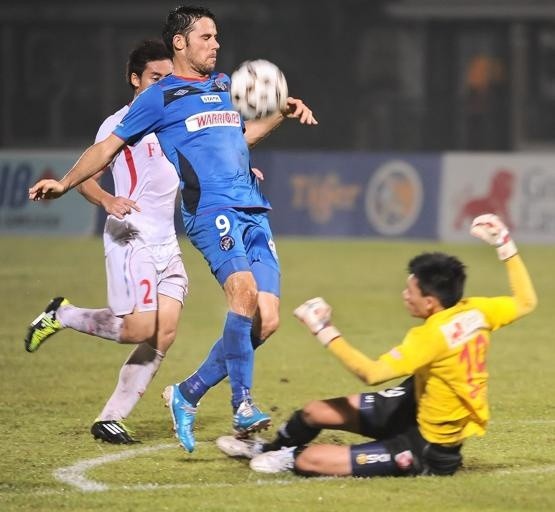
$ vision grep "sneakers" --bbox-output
[24,296,70,352]
[248,446,298,473]
[90,417,142,445]
[232,400,272,436]
[215,435,265,458]
[161,383,200,453]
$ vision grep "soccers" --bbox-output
[230,60,288,118]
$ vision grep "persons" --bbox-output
[217,213,539,477]
[24,39,263,444]
[29,4,318,452]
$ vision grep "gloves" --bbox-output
[293,297,341,348]
[470,214,518,261]
